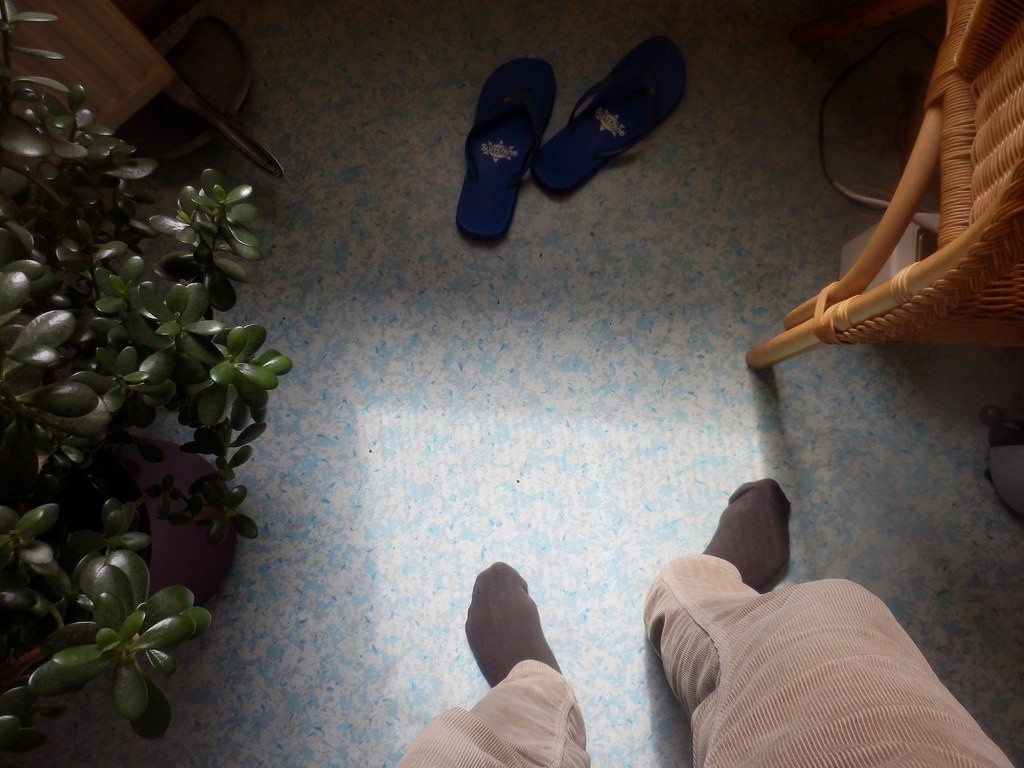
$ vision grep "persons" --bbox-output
[392,474,1013,768]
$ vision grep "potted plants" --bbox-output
[0,0,295,754]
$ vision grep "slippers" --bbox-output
[531,36,685,192]
[456,57,556,240]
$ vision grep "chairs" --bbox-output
[747,0,1024,382]
